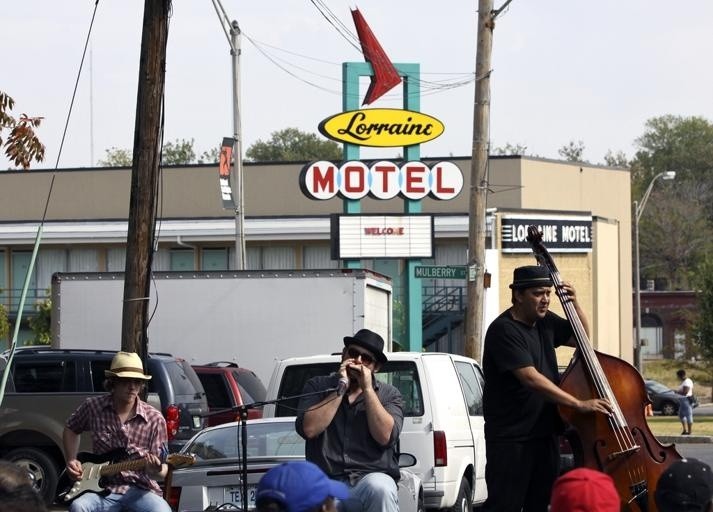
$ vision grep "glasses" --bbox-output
[348,347,372,365]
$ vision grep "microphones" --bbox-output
[336,377,350,396]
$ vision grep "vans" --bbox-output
[259,344,488,512]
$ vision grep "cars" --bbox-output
[170,412,425,511]
[644,377,699,416]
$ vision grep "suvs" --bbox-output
[0,343,210,511]
[189,361,267,425]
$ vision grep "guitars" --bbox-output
[56,451,196,506]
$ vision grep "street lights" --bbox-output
[633,169,678,374]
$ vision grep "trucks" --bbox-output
[1,267,393,387]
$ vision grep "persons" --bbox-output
[253,458,347,512]
[60,351,173,511]
[479,265,614,511]
[294,329,405,512]
[0,460,48,512]
[672,369,693,436]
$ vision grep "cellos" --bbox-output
[527,224,684,512]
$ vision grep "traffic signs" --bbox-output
[414,264,467,280]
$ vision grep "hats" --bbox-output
[105,352,152,380]
[344,329,387,363]
[510,266,554,288]
[257,462,351,511]
